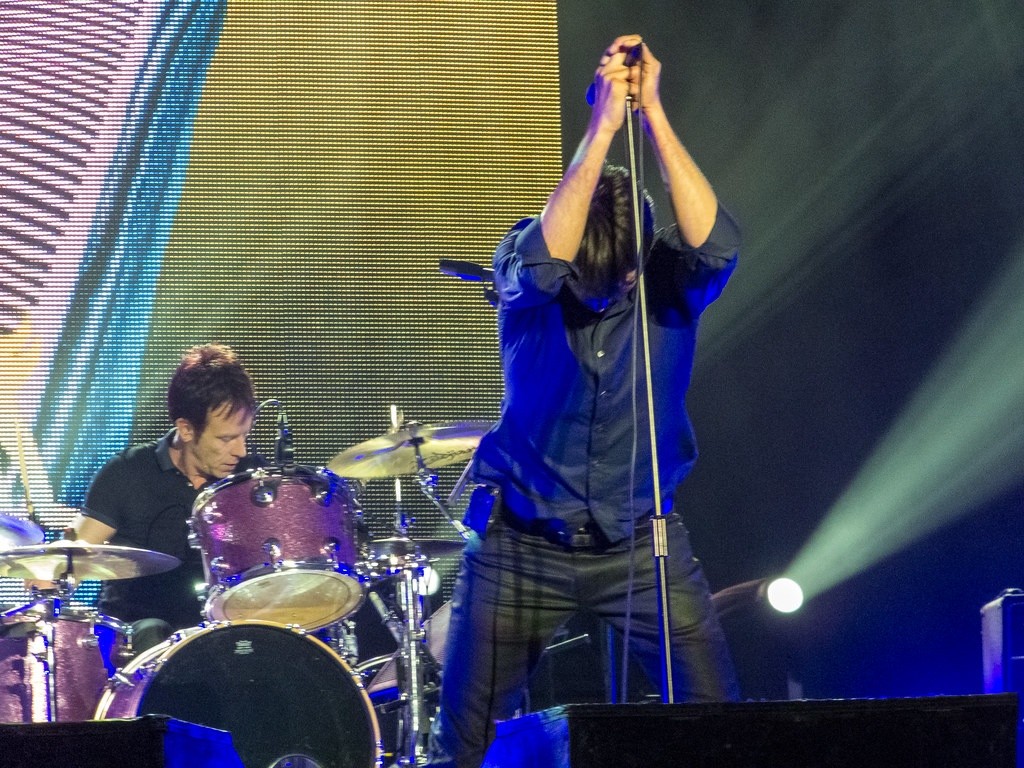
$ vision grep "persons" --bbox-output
[76,344,271,678]
[426,34,743,768]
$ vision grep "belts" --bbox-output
[500,504,651,548]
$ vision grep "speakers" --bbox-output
[480,694,1023,768]
[0,714,246,768]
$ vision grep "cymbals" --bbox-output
[0,512,45,546]
[327,418,498,479]
[366,532,470,560]
[0,529,182,581]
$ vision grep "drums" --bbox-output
[0,600,133,724]
[94,614,384,768]
[187,459,368,633]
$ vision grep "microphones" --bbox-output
[587,43,644,106]
[274,409,294,465]
